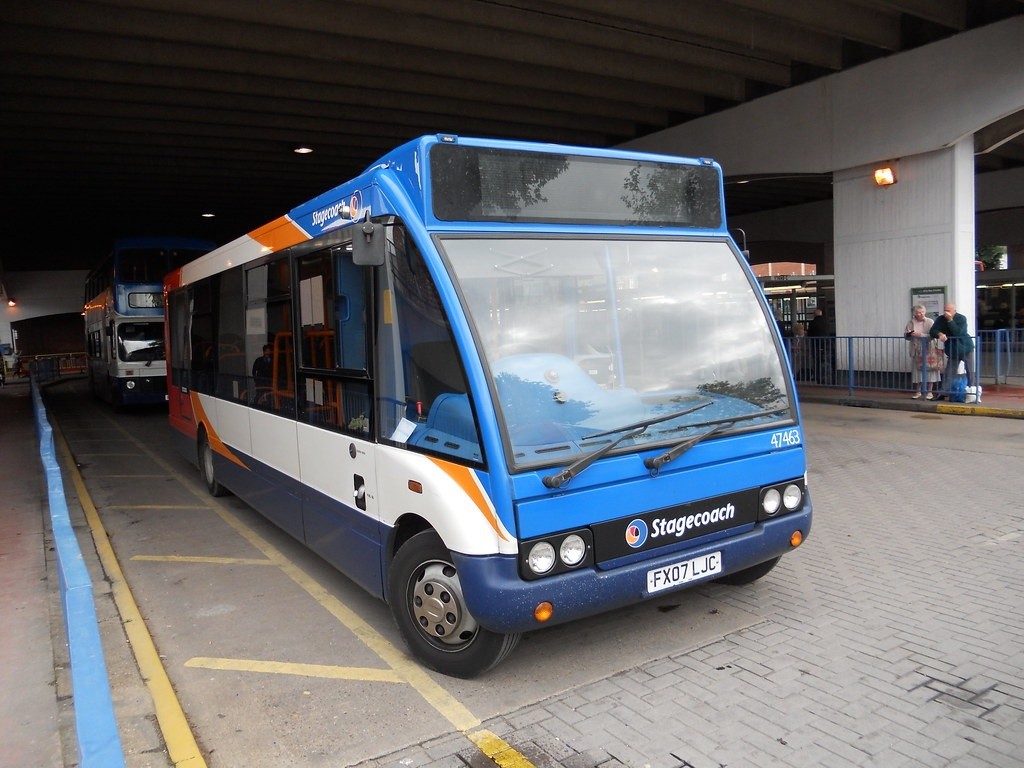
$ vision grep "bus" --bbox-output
[81,236,229,413]
[162,134,815,681]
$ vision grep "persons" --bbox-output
[995,301,1011,329]
[778,317,785,332]
[929,303,974,403]
[12,357,23,378]
[252,345,273,406]
[904,305,934,400]
[807,310,829,373]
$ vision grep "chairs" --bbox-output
[411,339,467,419]
[202,341,344,422]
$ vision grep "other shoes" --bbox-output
[932,394,946,401]
[926,394,933,399]
[911,393,921,399]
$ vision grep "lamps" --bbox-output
[874,166,898,185]
[8,298,16,306]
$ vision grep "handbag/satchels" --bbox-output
[918,339,944,370]
[965,385,982,403]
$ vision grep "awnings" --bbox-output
[976,269,1024,348]
